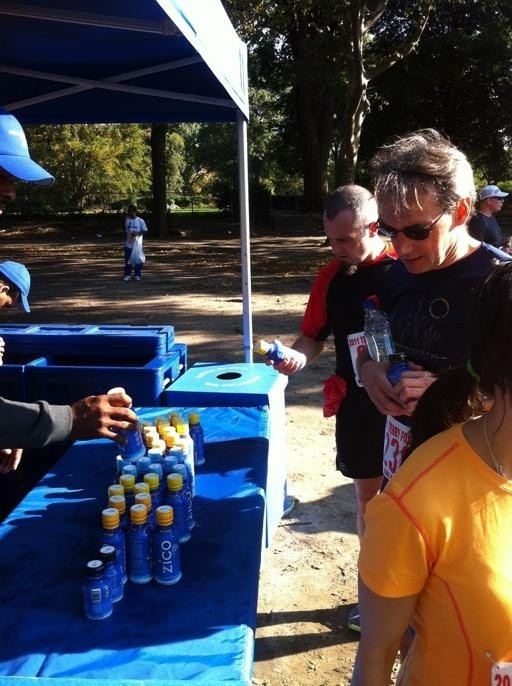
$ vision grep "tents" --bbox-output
[0,1,254,363]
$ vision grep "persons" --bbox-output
[349,262,512,686]
[122,205,148,282]
[265,182,400,634]
[467,184,512,256]
[0,111,140,476]
[349,128,512,488]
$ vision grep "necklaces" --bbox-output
[483,414,506,479]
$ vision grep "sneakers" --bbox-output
[134,276,141,281]
[122,276,132,281]
[348,603,363,633]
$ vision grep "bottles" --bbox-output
[147,448,164,467]
[99,507,128,584]
[368,294,392,364]
[162,426,176,441]
[133,482,150,502]
[177,422,194,470]
[253,340,306,372]
[99,547,124,603]
[126,503,153,584]
[167,433,180,456]
[113,455,131,485]
[162,456,179,481]
[144,431,159,451]
[172,417,184,433]
[157,421,171,440]
[152,439,167,458]
[362,300,396,367]
[107,387,146,461]
[107,485,130,508]
[163,473,192,543]
[143,426,157,442]
[152,505,182,584]
[141,422,152,438]
[188,413,206,466]
[148,463,166,494]
[388,352,412,409]
[119,474,136,505]
[169,448,193,484]
[135,493,156,530]
[143,473,162,507]
[122,464,140,486]
[169,413,179,426]
[82,560,113,621]
[172,464,195,530]
[155,417,169,430]
[109,495,128,531]
[137,455,151,481]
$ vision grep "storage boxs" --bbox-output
[0,361,26,402]
[24,333,188,393]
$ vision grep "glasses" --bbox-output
[373,202,452,241]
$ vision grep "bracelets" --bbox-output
[358,356,373,380]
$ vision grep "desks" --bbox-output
[0,404,277,685]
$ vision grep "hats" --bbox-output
[479,185,509,201]
[0,113,57,191]
